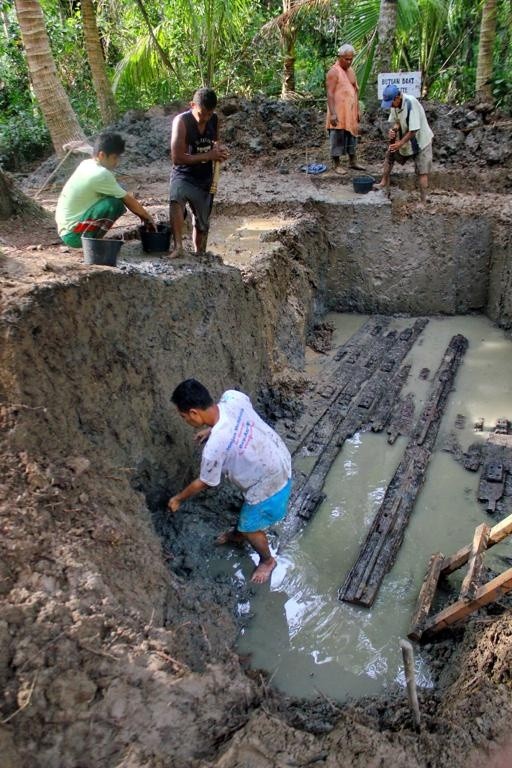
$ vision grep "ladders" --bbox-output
[407,513,512,645]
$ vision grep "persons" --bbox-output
[166,378,291,584]
[162,87,230,259]
[324,43,367,174]
[55,131,158,249]
[372,85,434,208]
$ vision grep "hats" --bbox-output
[380,84,399,110]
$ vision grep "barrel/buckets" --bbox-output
[80,218,124,267]
[138,223,172,253]
[351,177,376,194]
